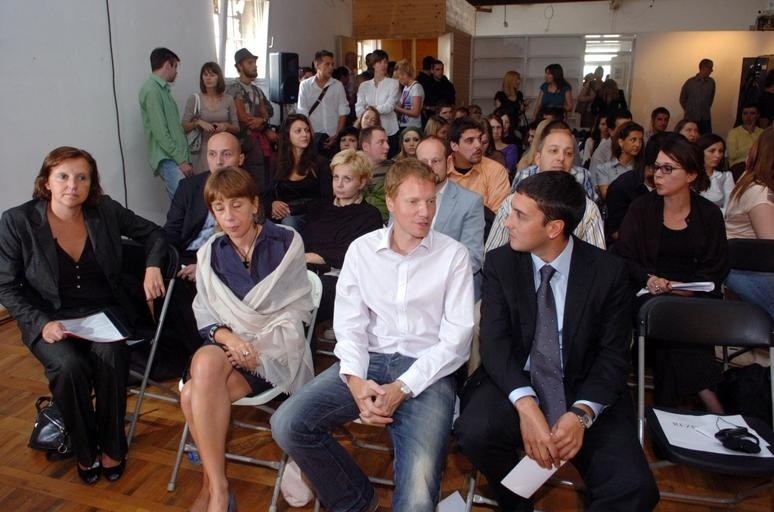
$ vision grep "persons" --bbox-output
[135,50,456,204]
[160,100,771,512]
[679,58,716,135]
[2,147,172,487]
[492,63,633,129]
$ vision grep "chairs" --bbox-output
[168,267,322,512]
[637,290,769,509]
[463,456,579,512]
[727,237,772,318]
[337,415,394,489]
[119,239,181,450]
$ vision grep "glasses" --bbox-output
[649,162,687,175]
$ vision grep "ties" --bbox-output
[529,264,568,427]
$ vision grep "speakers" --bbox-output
[270,51,299,104]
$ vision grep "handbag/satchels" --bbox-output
[26,396,73,454]
[187,93,202,153]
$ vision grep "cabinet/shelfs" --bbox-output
[468,34,586,127]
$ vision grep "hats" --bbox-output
[234,47,259,66]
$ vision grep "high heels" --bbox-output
[100,445,127,483]
[75,454,101,488]
